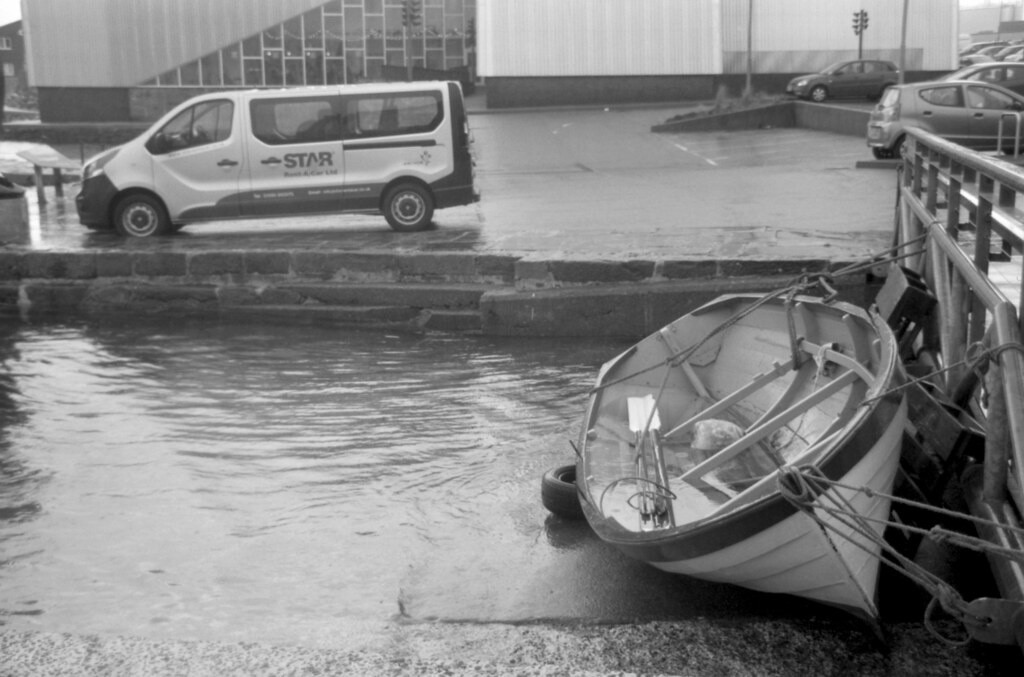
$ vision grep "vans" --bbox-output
[74,74,483,238]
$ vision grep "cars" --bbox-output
[786,57,900,103]
[932,38,1024,96]
[867,79,1024,160]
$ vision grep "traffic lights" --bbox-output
[852,12,861,35]
[862,11,869,29]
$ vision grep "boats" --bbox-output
[571,289,909,621]
